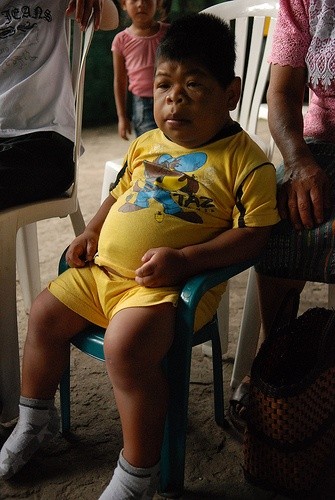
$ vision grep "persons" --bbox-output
[110,0,169,140]
[0,0,120,215]
[228,0,335,427]
[0,12,281,500]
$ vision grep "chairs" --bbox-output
[231,260,335,394]
[197,1,283,167]
[59,244,226,496]
[1,10,95,424]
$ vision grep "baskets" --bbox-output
[243,307,335,494]
